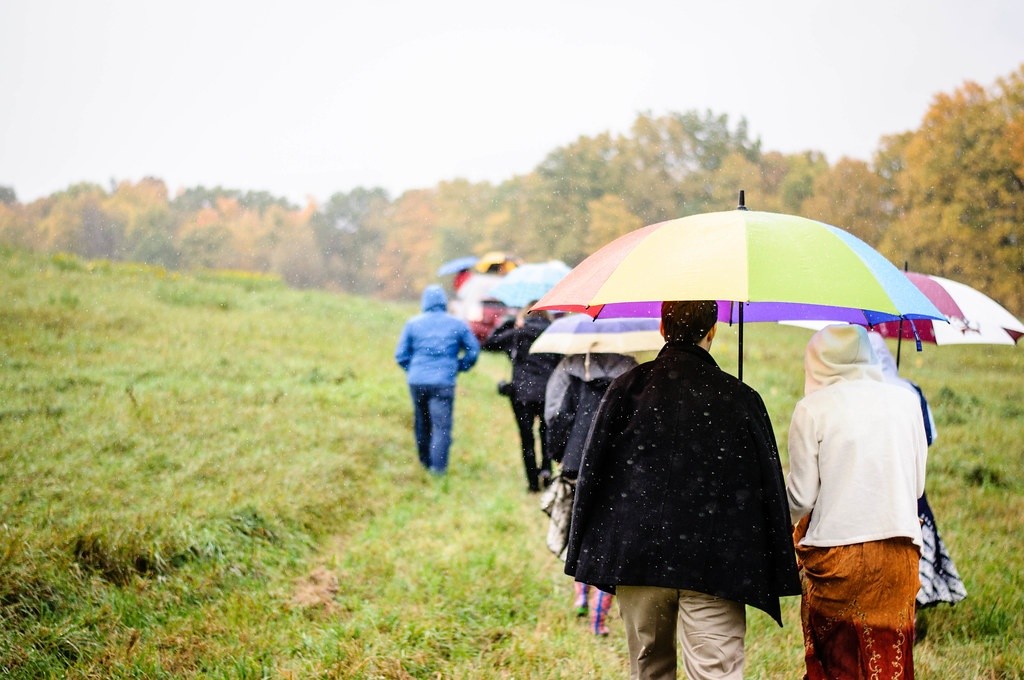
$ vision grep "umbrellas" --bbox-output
[440,189,1024,381]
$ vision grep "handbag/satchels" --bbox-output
[541,480,577,556]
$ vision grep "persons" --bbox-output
[398,262,938,680]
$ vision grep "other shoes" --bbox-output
[575,605,589,619]
